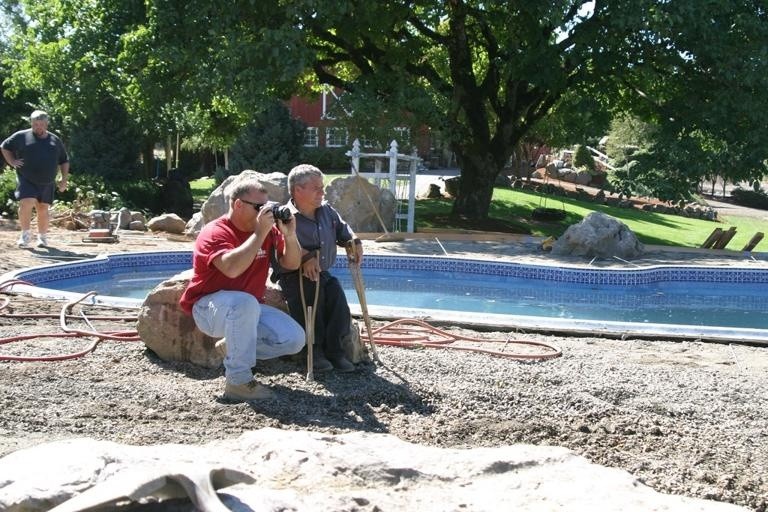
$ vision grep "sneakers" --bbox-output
[37,237,47,246]
[306,347,333,374]
[223,379,275,399]
[331,354,354,371]
[215,337,228,359]
[17,232,31,245]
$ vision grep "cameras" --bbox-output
[259,201,290,219]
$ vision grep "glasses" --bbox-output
[232,198,263,210]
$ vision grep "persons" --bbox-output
[180,178,306,401]
[0,110,69,246]
[270,163,363,372]
